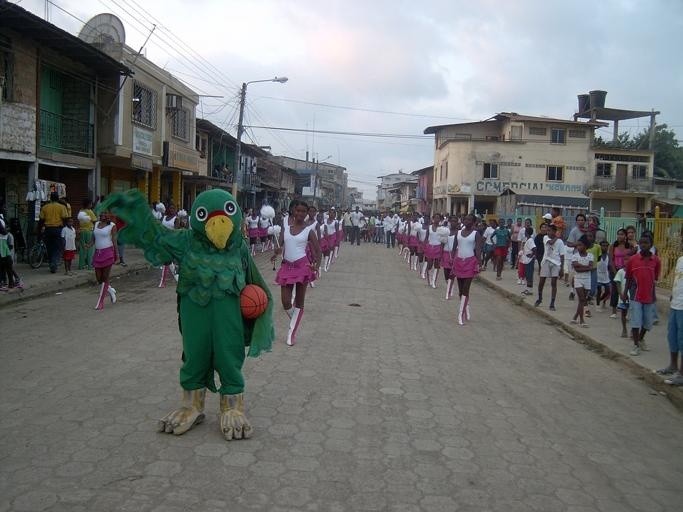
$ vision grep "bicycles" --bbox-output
[27,227,64,268]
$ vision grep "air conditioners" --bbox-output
[166,94,182,109]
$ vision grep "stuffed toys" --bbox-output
[95,187,276,440]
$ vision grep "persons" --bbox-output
[425,213,447,289]
[241,207,289,257]
[1,210,25,290]
[114,239,128,267]
[542,213,553,226]
[521,228,537,296]
[303,206,344,288]
[534,226,566,312]
[92,209,120,311]
[439,216,459,299]
[150,199,192,288]
[450,214,482,326]
[395,213,431,278]
[271,201,322,346]
[550,207,565,239]
[61,218,78,276]
[534,223,547,276]
[77,199,98,269]
[439,214,535,286]
[567,213,660,356]
[38,191,69,273]
[656,257,682,385]
[343,206,402,248]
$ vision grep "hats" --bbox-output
[542,214,553,220]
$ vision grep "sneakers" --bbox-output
[568,292,618,327]
[621,327,650,356]
[653,364,683,385]
[550,302,556,312]
[516,278,533,296]
[534,299,542,306]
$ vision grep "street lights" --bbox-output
[230,74,289,199]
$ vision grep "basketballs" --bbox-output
[239,283,267,319]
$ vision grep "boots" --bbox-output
[250,239,274,256]
[159,262,179,288]
[399,244,470,325]
[95,282,116,311]
[284,246,338,346]
